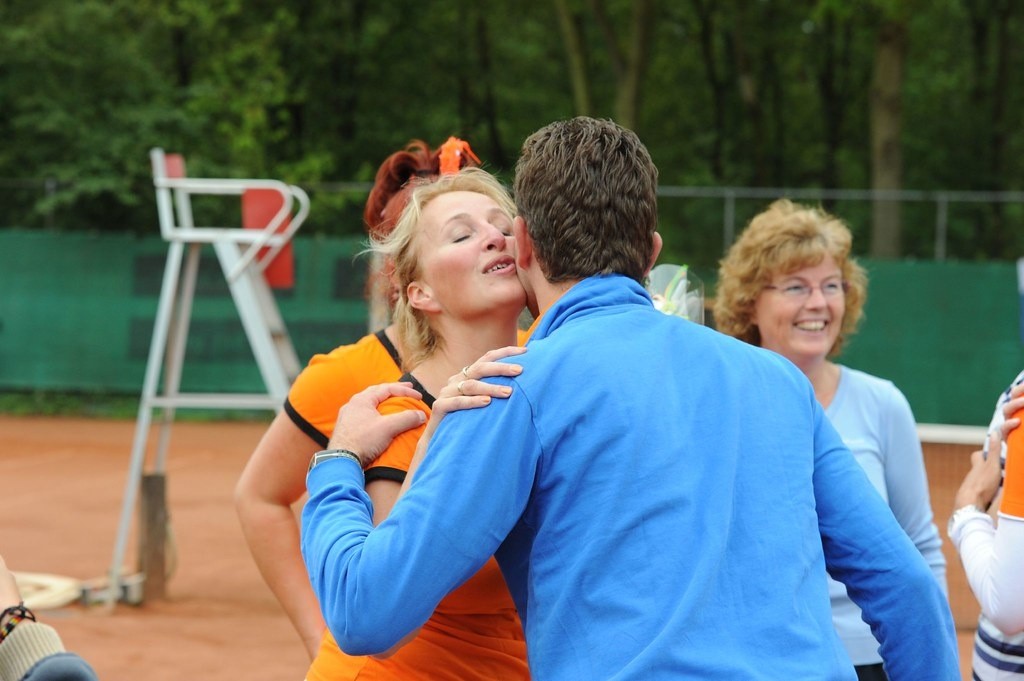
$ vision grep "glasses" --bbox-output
[764,274,846,300]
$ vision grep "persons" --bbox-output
[294,116,961,680]
[306,163,532,679]
[0,550,98,681]
[712,194,954,679]
[947,380,1024,640]
[234,131,529,680]
[947,363,1024,681]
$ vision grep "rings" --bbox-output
[462,365,473,378]
[458,380,468,395]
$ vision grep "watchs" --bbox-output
[305,448,364,485]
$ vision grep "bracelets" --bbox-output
[0,600,35,645]
